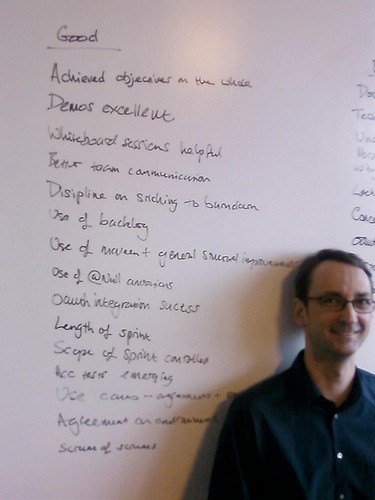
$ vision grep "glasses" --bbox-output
[303,295,375,314]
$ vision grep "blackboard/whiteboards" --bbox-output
[2,0,374,499]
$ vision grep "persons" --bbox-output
[206,248,375,500]
